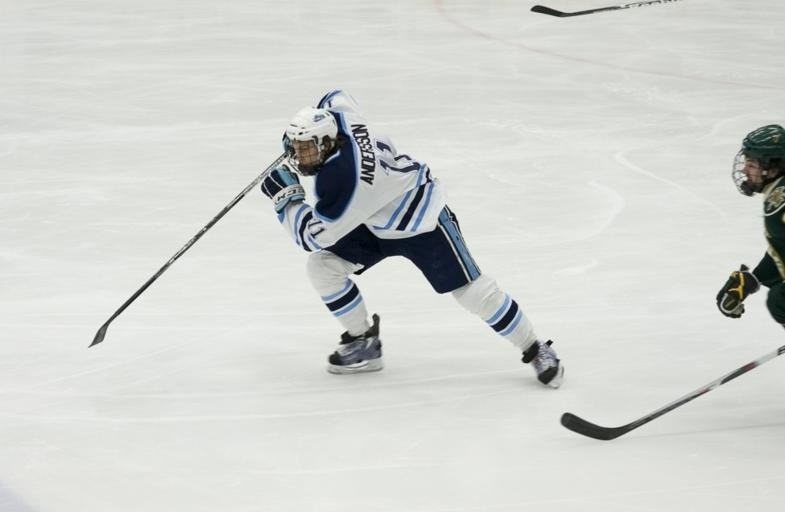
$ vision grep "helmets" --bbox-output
[732,123,784,196]
[282,106,338,176]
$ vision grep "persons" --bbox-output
[260,88,566,389]
[716,124,785,327]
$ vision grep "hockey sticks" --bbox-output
[89,149,291,345]
[562,343,785,440]
[530,1,676,18]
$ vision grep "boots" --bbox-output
[329,313,383,366]
[521,339,558,385]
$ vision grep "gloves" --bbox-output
[717,265,761,318]
[261,165,305,213]
